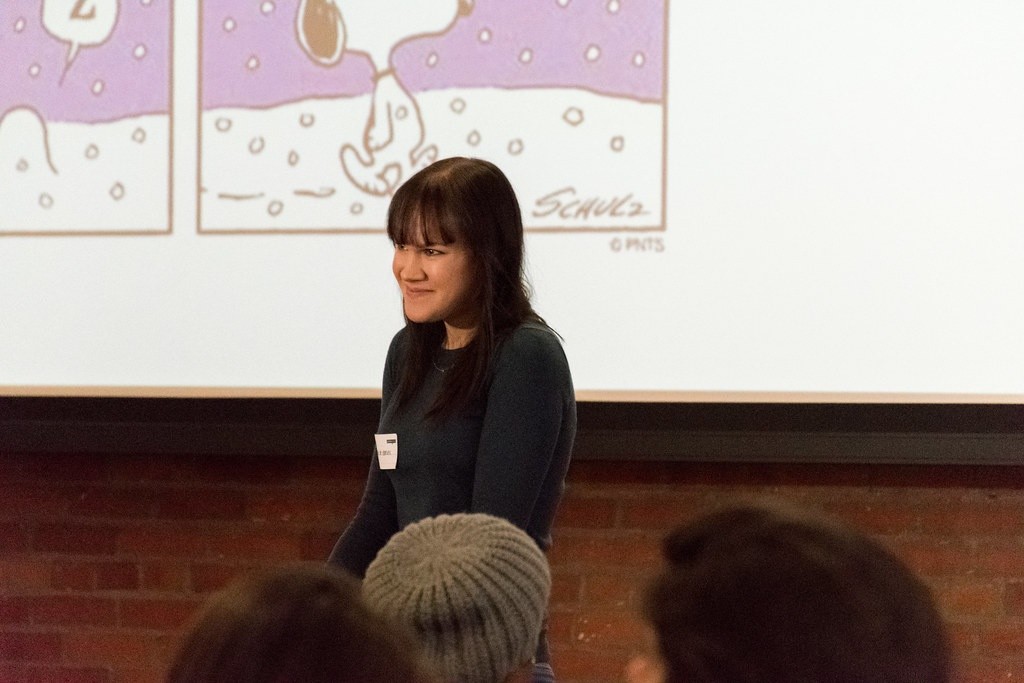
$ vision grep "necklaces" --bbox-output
[431,353,454,372]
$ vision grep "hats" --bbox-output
[359,514,552,683]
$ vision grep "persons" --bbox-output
[161,511,952,682]
[320,156,578,682]
